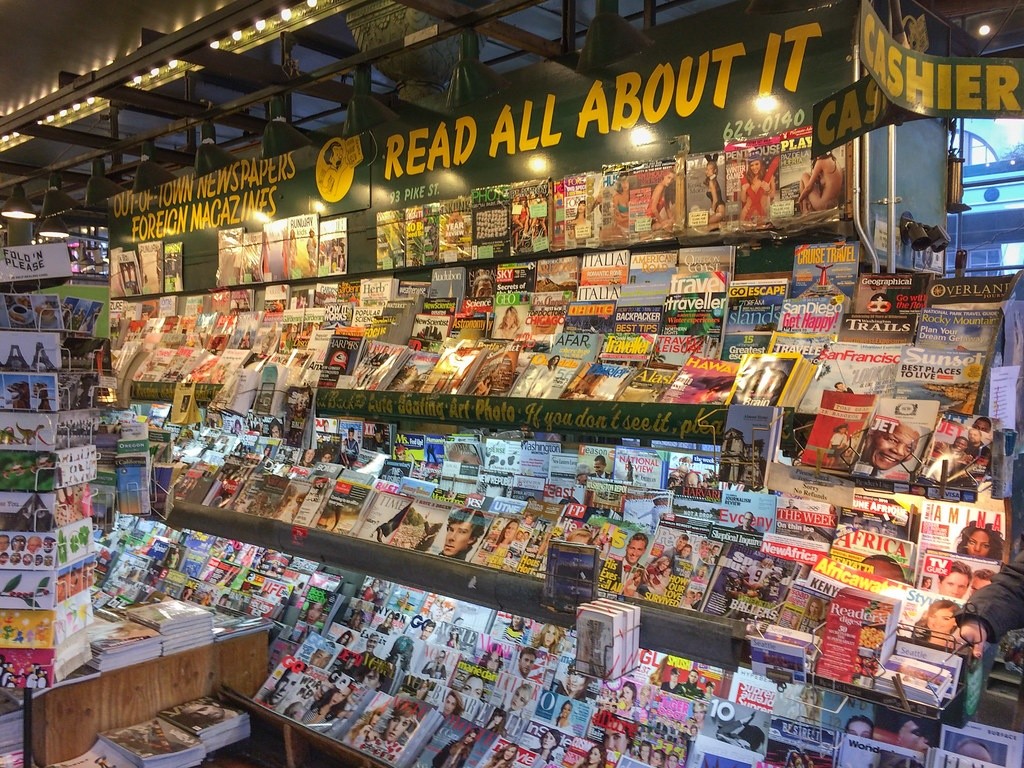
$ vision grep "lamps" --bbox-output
[900,148,972,253]
[0,0,660,238]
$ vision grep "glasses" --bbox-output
[397,451,405,456]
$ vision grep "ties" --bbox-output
[435,666,439,672]
[624,565,631,572]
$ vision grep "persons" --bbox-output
[361,701,419,763]
[485,743,519,768]
[939,560,972,600]
[927,601,961,649]
[589,456,610,479]
[966,429,992,490]
[846,714,874,740]
[440,508,485,561]
[950,550,1024,658]
[734,511,756,532]
[859,422,922,479]
[787,686,823,726]
[182,701,245,727]
[345,427,359,463]
[602,532,649,595]
[834,381,853,394]
[957,520,1004,561]
[972,417,992,433]
[950,436,969,476]
[971,570,997,593]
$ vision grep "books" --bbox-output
[0,124,1024,768]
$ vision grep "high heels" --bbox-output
[374,526,383,542]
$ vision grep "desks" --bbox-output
[24,630,268,766]
